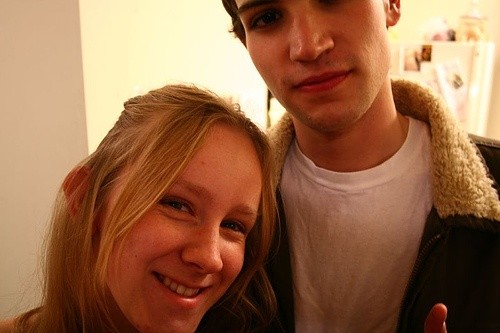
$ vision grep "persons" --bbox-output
[221,0,500,333]
[0,85,281,333]
